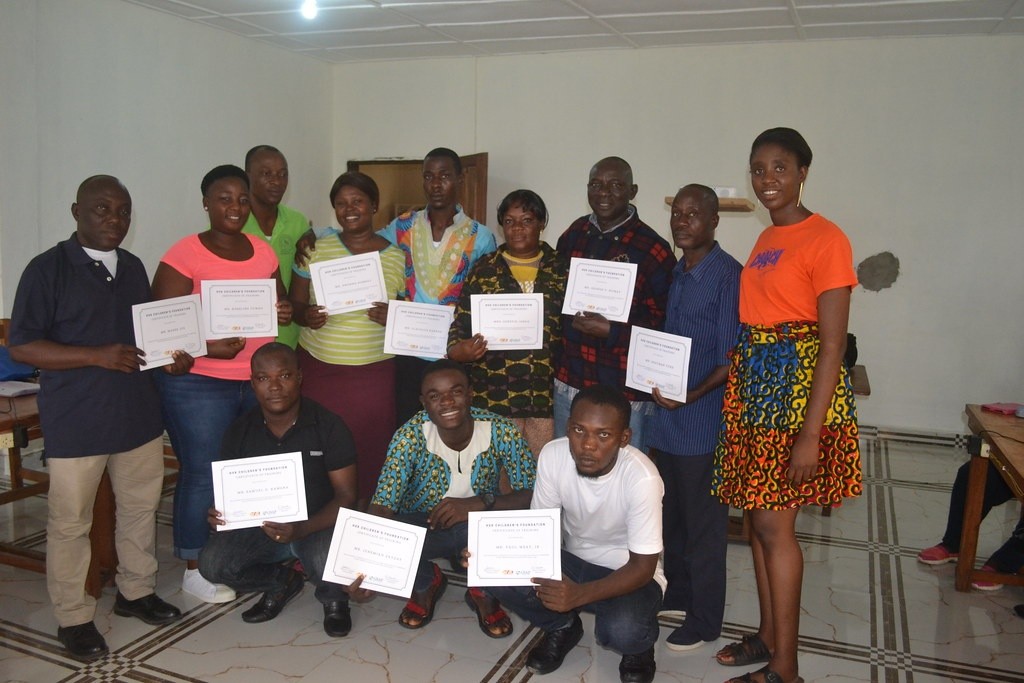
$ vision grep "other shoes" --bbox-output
[666,625,708,651]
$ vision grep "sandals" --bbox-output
[399,562,447,628]
[465,587,513,638]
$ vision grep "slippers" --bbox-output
[725,662,804,683]
[716,635,770,666]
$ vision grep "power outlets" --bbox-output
[0,433,14,449]
[980,438,990,457]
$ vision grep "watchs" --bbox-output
[481,494,495,509]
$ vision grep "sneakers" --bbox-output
[917,543,959,565]
[182,569,236,603]
[971,564,1004,592]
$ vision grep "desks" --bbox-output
[0,375,118,598]
[955,405,1024,592]
[821,365,871,516]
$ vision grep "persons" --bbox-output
[718,127,861,683]
[460,384,666,683]
[294,147,496,309]
[552,156,677,453]
[918,460,1024,590]
[198,342,356,637]
[446,190,568,494]
[341,358,535,638]
[8,175,194,661]
[647,184,744,649]
[290,173,407,506]
[204,145,311,350]
[153,165,295,603]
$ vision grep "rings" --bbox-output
[276,536,280,540]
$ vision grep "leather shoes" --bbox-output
[619,644,656,683]
[242,570,304,623]
[324,598,351,637]
[526,614,583,676]
[113,589,181,624]
[58,620,109,659]
